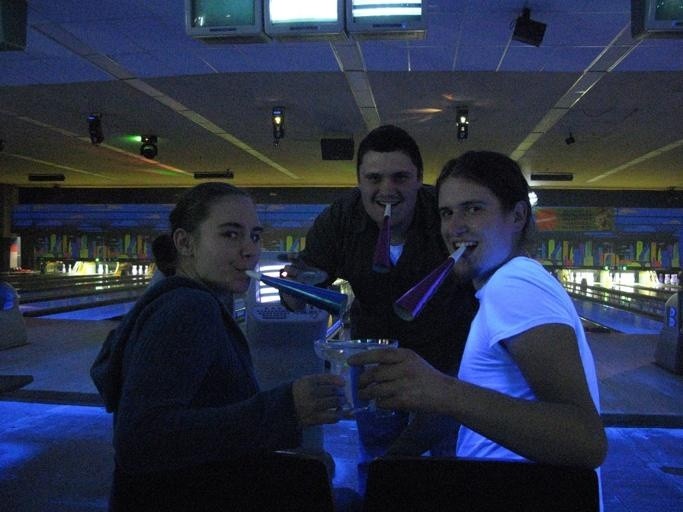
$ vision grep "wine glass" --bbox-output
[314,336,401,422]
[289,263,327,316]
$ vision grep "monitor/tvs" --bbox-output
[344,0,427,42]
[630,0,683,40]
[185,0,273,46]
[262,0,344,42]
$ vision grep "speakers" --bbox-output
[320,137,355,161]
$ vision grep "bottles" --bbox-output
[535,237,683,290]
[33,230,156,275]
[277,234,308,252]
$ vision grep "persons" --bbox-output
[276,125,479,496]
[346,149,608,512]
[89,183,348,511]
[0,277,29,353]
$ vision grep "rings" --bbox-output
[313,379,321,390]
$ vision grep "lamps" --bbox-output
[86,113,104,146]
[271,107,286,140]
[454,109,471,146]
[137,135,159,159]
